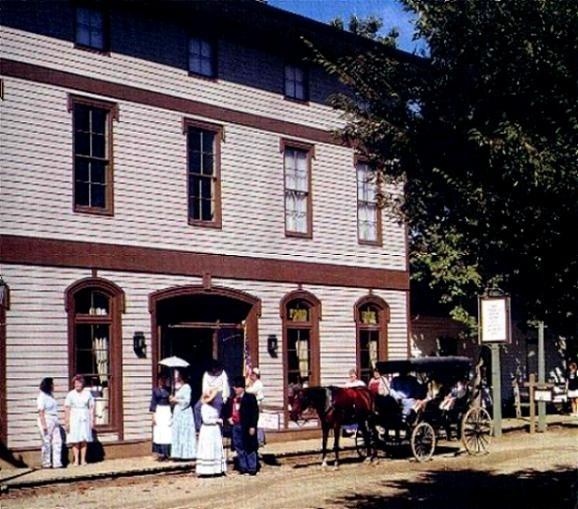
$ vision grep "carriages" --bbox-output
[289,356,494,469]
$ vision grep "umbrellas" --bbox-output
[157,354,191,395]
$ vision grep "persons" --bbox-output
[65,373,97,467]
[36,378,66,469]
[146,360,267,479]
[342,366,470,440]
[564,361,578,418]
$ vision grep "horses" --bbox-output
[285,384,382,471]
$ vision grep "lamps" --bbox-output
[134,331,146,350]
[268,334,277,350]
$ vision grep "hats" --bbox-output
[232,376,248,388]
[252,368,261,377]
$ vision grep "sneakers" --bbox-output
[73,462,88,467]
[155,456,169,462]
